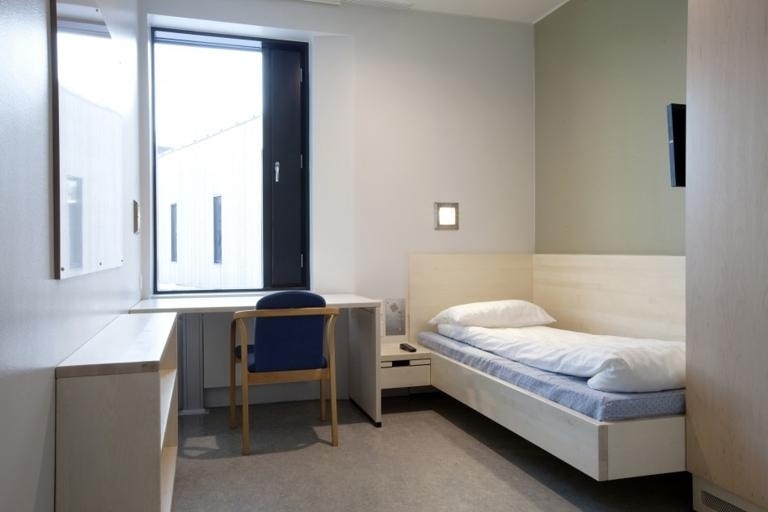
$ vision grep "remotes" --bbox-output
[400,342,416,352]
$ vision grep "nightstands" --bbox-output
[380,342,432,389]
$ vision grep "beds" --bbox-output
[404,252,686,483]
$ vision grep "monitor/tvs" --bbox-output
[665,103,686,187]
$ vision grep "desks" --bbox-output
[128,291,385,428]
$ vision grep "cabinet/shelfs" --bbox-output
[54,312,178,511]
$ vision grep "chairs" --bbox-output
[227,291,340,456]
